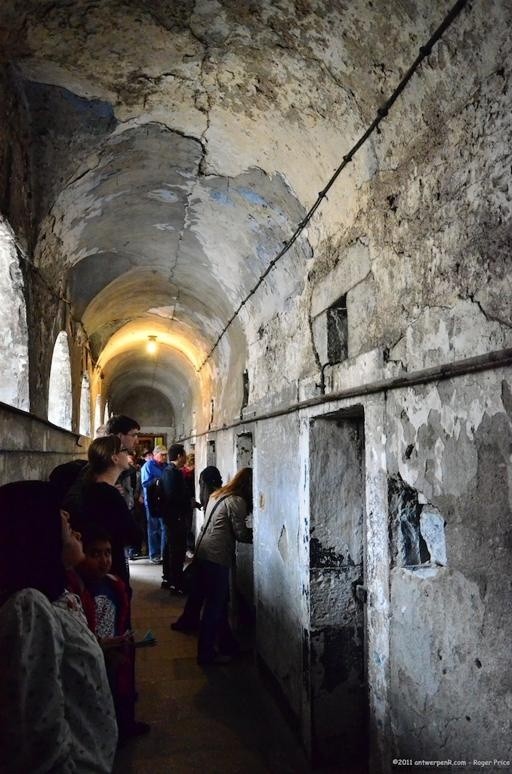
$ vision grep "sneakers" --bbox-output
[170,622,200,632]
[150,557,161,565]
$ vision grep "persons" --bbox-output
[135,444,254,662]
[63,521,132,675]
[67,412,140,579]
[0,475,118,774]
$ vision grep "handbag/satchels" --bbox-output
[179,562,204,597]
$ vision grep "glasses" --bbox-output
[115,448,128,454]
